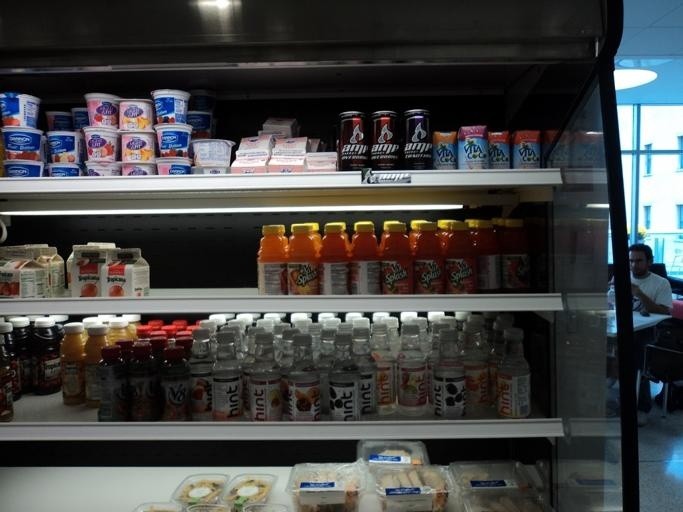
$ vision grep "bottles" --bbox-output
[0,311,536,421]
[258,219,531,294]
[608,284,617,336]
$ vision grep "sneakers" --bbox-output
[638,411,648,427]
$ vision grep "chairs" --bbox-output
[643,299,683,416]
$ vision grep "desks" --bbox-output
[606,310,671,426]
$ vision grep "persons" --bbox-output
[607,243,674,416]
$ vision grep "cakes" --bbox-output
[179,479,225,506]
[226,479,272,506]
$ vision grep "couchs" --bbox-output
[608,263,683,295]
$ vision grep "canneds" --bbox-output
[336,108,433,171]
